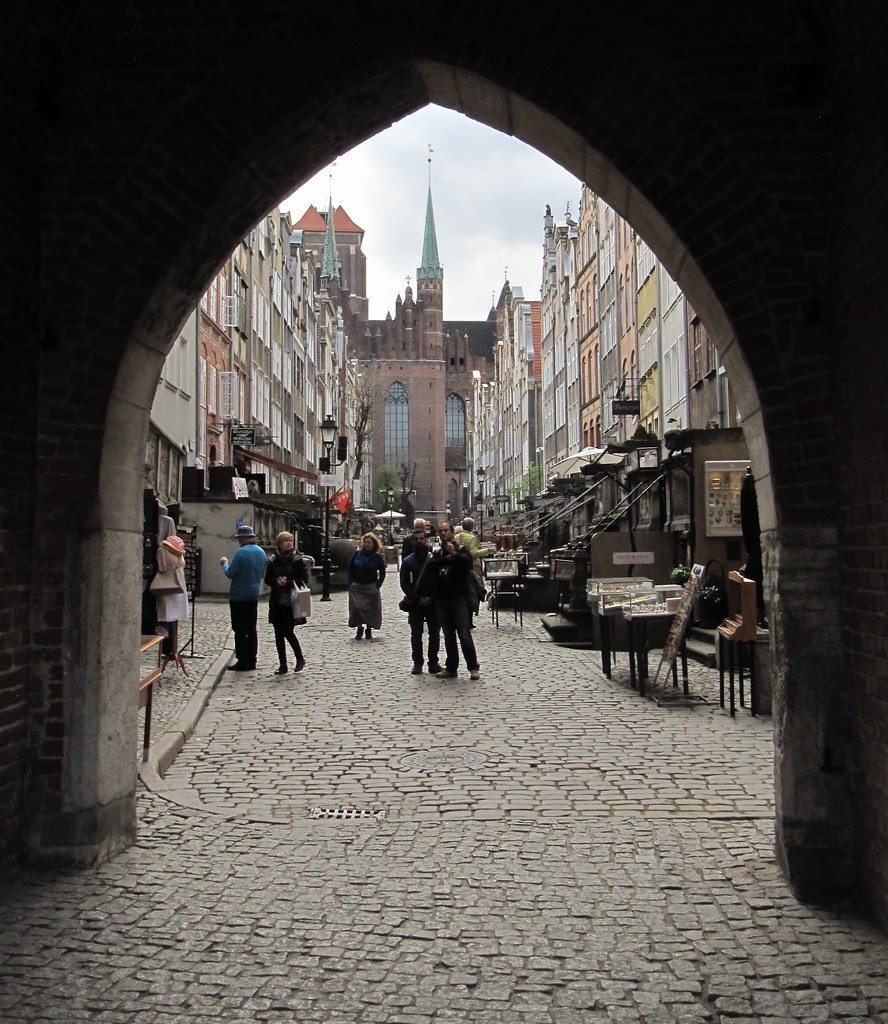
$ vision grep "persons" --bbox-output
[265,531,309,675]
[220,525,266,672]
[347,532,386,640]
[400,517,497,680]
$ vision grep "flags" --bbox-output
[324,487,352,514]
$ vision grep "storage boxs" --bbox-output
[554,559,575,580]
[624,446,658,474]
[587,578,685,619]
[482,552,530,579]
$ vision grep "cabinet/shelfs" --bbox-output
[486,579,524,631]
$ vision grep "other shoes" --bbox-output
[274,663,288,674]
[435,670,457,677]
[411,660,422,674]
[365,629,373,639]
[294,659,305,673]
[428,664,444,673]
[355,626,364,639]
[470,669,478,679]
[227,659,256,671]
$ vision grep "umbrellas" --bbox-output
[550,445,627,476]
[375,510,405,518]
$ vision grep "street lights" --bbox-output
[476,466,485,543]
[447,500,451,520]
[321,414,337,602]
[387,484,396,525]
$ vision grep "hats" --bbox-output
[163,536,186,554]
[233,526,256,539]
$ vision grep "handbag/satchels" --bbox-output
[399,596,416,612]
[291,578,311,618]
[149,567,186,595]
